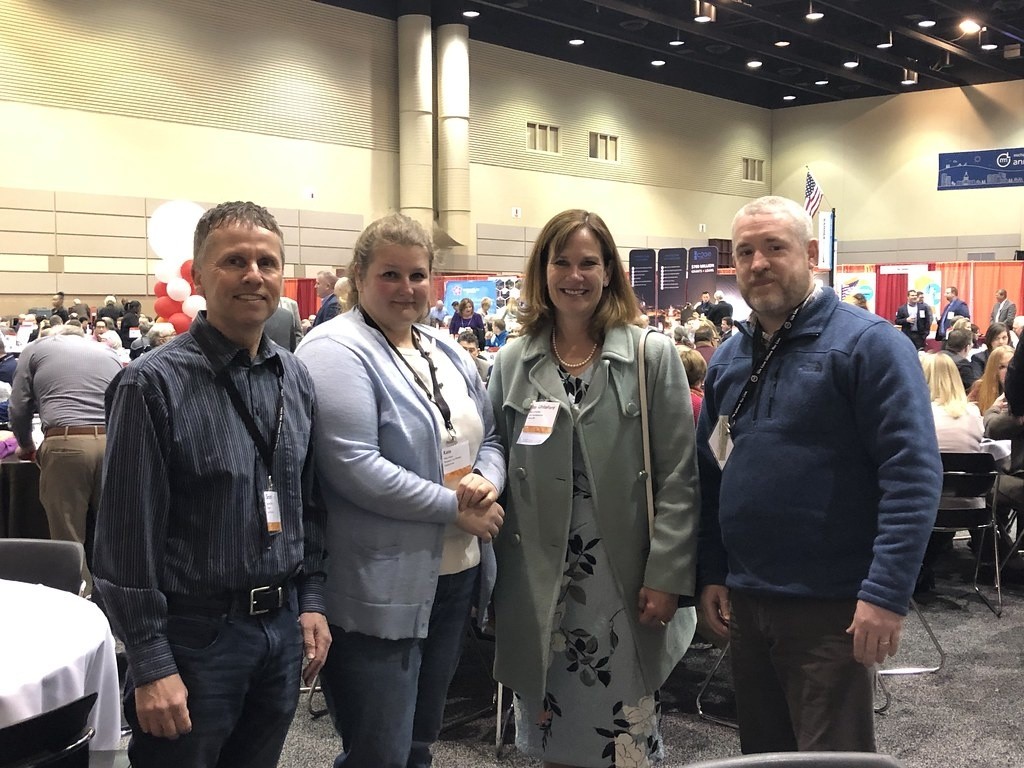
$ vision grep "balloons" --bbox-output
[149,201,208,335]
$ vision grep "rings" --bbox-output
[879,640,891,647]
[659,619,668,626]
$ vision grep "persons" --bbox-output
[0,292,174,598]
[697,196,943,754]
[431,297,519,388]
[854,286,1024,595]
[293,214,507,768]
[92,201,331,768]
[488,209,696,768]
[640,291,733,421]
[265,271,350,352]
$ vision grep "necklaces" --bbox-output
[553,327,598,368]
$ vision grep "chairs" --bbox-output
[0,404,1024,768]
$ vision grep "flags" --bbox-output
[804,173,823,216]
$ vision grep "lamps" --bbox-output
[463,0,998,100]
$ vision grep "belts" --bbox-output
[161,584,289,615]
[44,426,106,437]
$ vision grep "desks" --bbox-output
[0,579,122,751]
[0,412,101,570]
